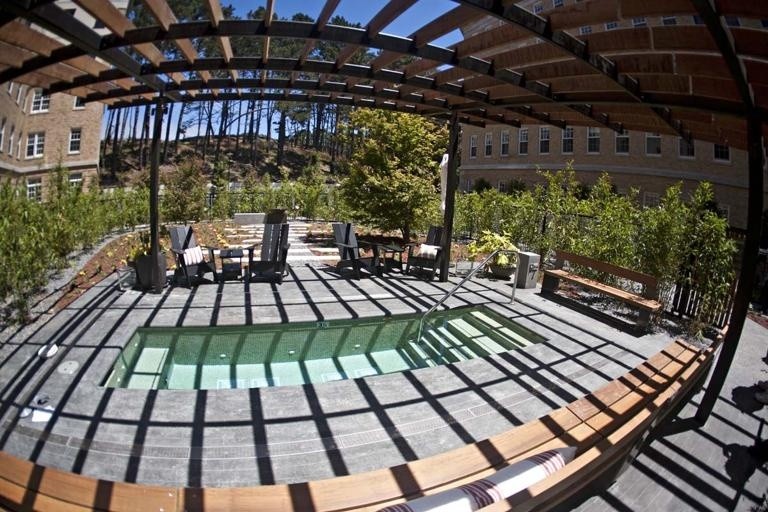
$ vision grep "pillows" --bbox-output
[373,446,575,512]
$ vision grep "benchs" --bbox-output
[2,324,728,512]
[539,248,665,336]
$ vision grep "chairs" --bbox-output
[333,222,442,281]
[164,223,290,285]
